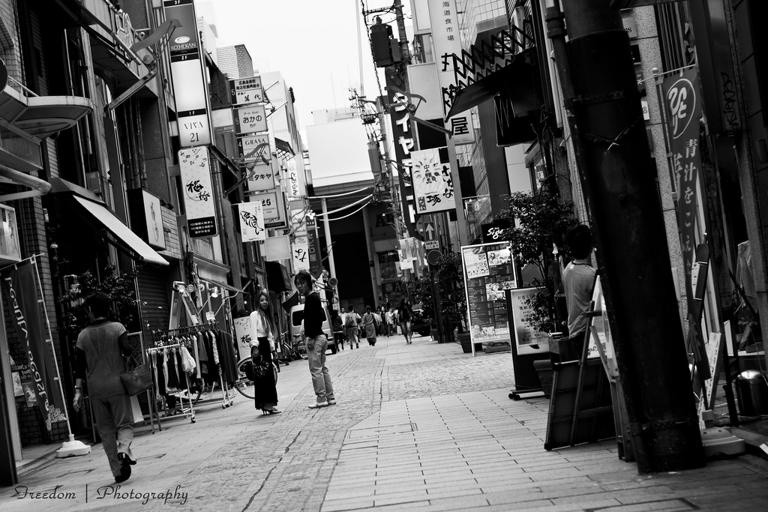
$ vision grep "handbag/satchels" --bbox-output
[119,364,154,397]
[245,361,273,383]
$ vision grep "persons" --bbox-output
[331,293,423,350]
[558,222,601,359]
[70,292,140,484]
[246,290,285,418]
[291,269,338,410]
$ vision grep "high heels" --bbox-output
[262,406,282,416]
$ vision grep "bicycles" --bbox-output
[234,330,308,399]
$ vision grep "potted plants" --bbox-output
[492,189,581,400]
[411,247,484,353]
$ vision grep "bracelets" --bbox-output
[73,385,81,390]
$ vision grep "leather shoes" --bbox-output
[308,399,336,408]
[115,453,131,483]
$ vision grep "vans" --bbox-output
[290,302,336,354]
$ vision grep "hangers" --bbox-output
[167,317,223,342]
